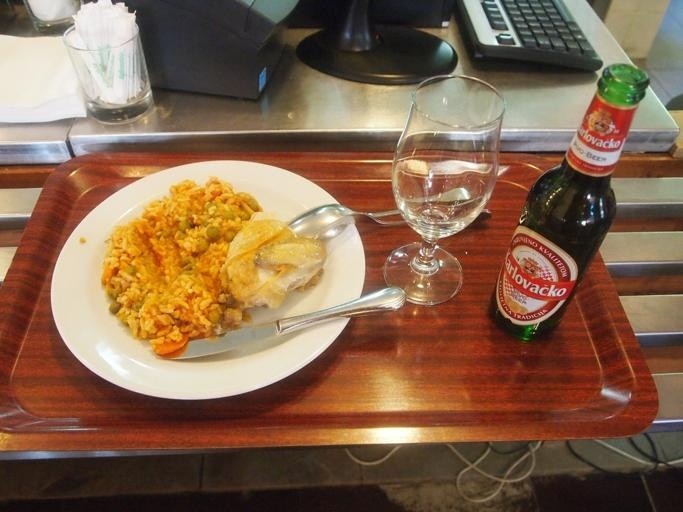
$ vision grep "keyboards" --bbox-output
[458,0,600,73]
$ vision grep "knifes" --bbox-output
[164,289,403,360]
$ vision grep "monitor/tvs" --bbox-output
[291,1,456,84]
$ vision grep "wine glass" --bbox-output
[380,74,505,309]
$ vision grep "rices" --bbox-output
[102,178,261,351]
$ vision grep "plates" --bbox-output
[45,161,364,401]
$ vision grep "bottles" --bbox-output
[487,61,648,340]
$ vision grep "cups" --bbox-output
[64,24,154,124]
[24,1,86,34]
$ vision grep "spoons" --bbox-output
[285,204,494,243]
